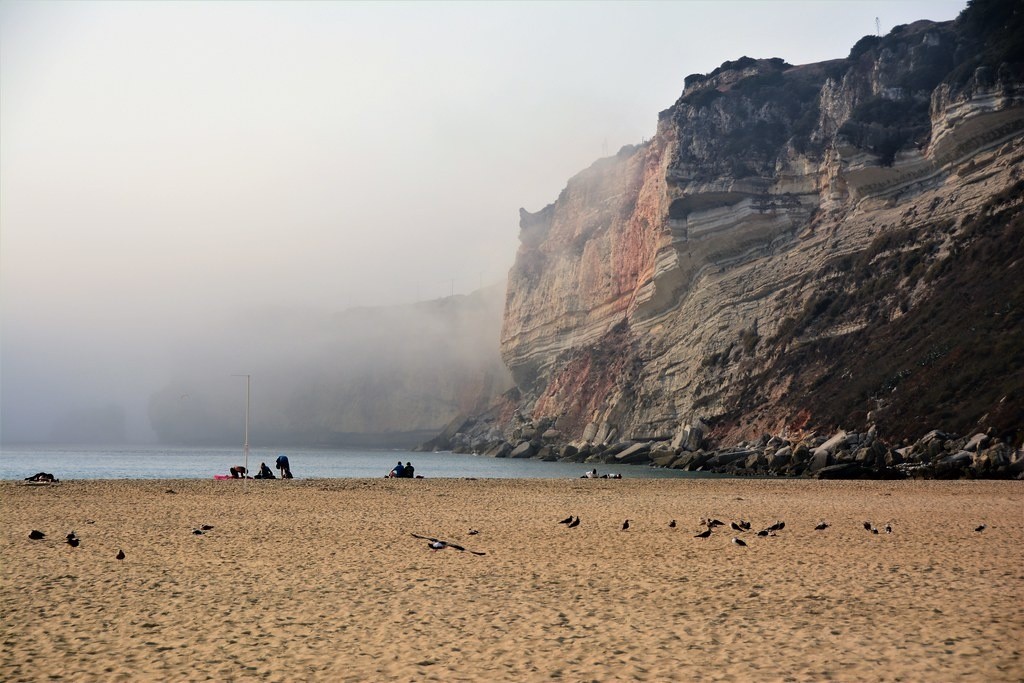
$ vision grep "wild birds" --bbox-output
[191,524,214,536]
[862,521,892,535]
[409,531,486,556]
[28,528,49,541]
[115,548,126,565]
[692,516,786,550]
[61,530,82,550]
[620,519,630,532]
[667,519,677,530]
[558,514,581,529]
[974,523,988,535]
[813,522,830,532]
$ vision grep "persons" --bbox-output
[230,466,248,478]
[401,462,414,478]
[276,456,293,479]
[389,461,405,478]
[585,469,599,478]
[601,473,622,479]
[258,462,272,478]
